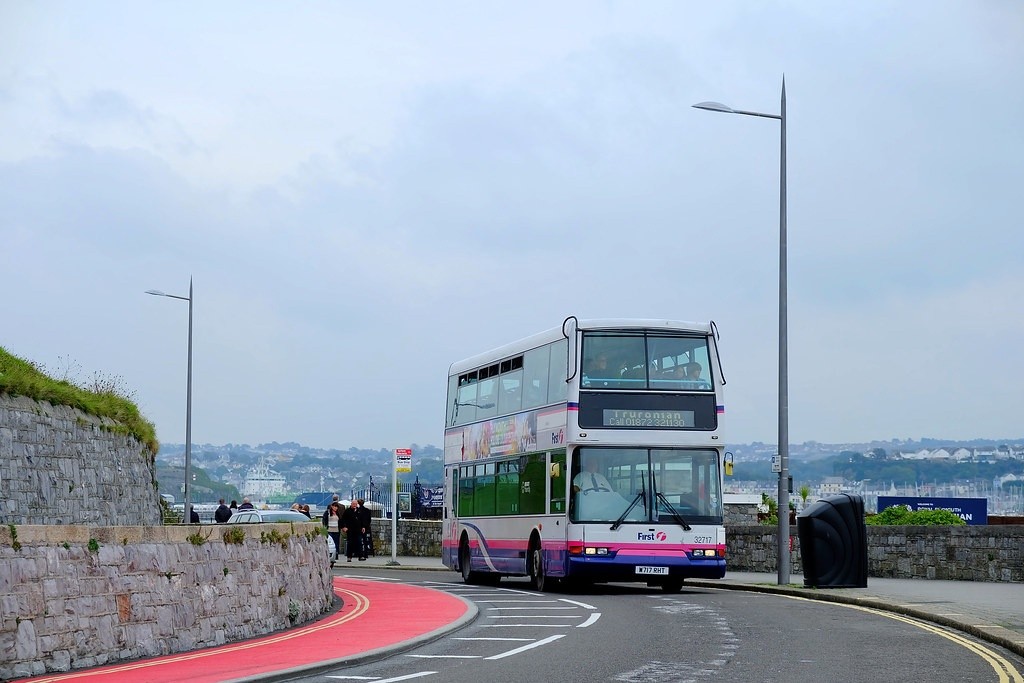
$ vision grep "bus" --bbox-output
[442,316,734,594]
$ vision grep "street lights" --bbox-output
[691,100,793,583]
[143,288,193,524]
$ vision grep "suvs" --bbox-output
[228,508,337,570]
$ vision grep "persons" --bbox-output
[322,502,346,560]
[573,456,614,493]
[289,503,311,520]
[341,499,371,562]
[238,497,254,510]
[182,504,200,523]
[214,499,233,523]
[330,496,345,511]
[584,352,711,389]
[229,500,238,515]
